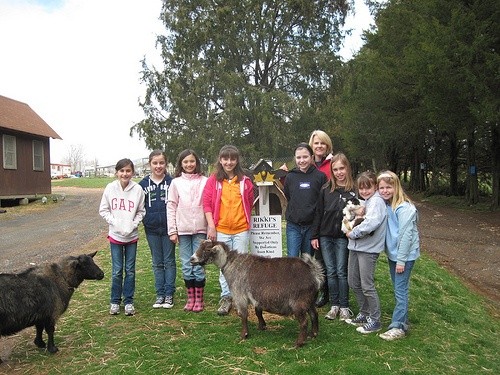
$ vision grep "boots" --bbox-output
[183,278,196,310]
[192,278,206,312]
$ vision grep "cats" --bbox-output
[343,195,373,238]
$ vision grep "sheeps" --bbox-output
[1,252,103,367]
[188,237,327,347]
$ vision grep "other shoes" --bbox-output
[315,298,327,307]
[218,297,232,315]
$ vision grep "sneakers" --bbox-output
[345,313,369,326]
[125,303,135,315]
[339,307,354,320]
[109,303,120,314]
[378,327,405,341]
[153,295,165,308]
[356,315,382,333]
[325,305,339,319]
[163,296,175,308]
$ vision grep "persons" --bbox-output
[99,158,147,315]
[166,149,210,312]
[343,170,388,334]
[283,143,327,260]
[139,150,176,308]
[310,152,359,322]
[375,170,421,340]
[202,145,255,317]
[306,130,336,306]
